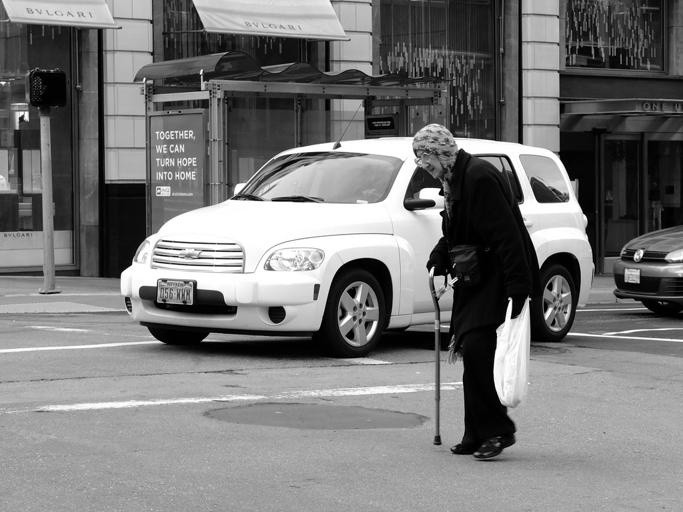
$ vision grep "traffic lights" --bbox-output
[26,68,67,108]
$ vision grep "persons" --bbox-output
[411,122,540,460]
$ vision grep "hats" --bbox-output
[412,123,458,170]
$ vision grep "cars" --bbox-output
[610,223,683,317]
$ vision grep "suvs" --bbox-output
[115,130,594,356]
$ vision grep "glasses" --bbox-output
[413,151,432,168]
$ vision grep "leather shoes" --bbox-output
[450,444,469,454]
[473,434,514,458]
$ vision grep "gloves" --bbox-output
[502,295,526,317]
[425,253,449,276]
